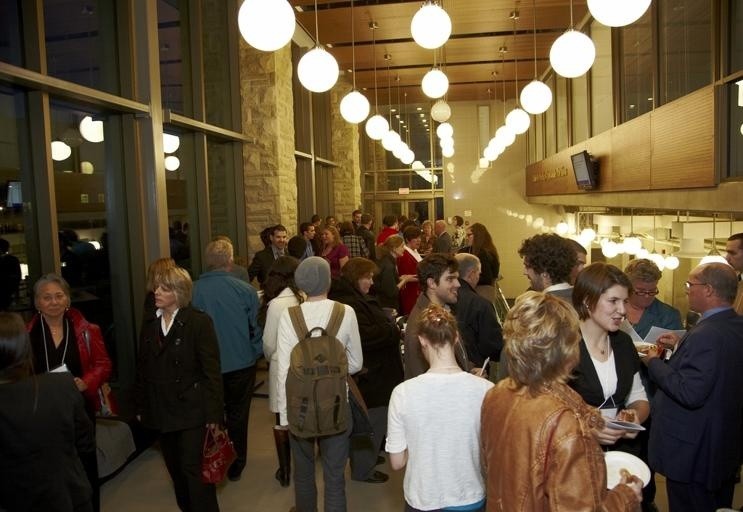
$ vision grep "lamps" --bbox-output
[495,126,515,146]
[587,0,651,27]
[440,137,454,149]
[297,48,339,93]
[489,138,505,155]
[601,237,679,272]
[366,115,390,139]
[699,256,729,266]
[442,148,454,157]
[550,31,596,78]
[410,5,452,50]
[340,91,370,123]
[484,147,498,161]
[556,222,596,250]
[430,102,452,122]
[505,109,530,135]
[437,123,453,138]
[520,81,552,114]
[237,0,297,53]
[422,69,449,99]
[401,149,415,165]
[393,141,408,159]
[383,131,401,151]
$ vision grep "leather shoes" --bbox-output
[375,456,385,464]
[361,471,389,483]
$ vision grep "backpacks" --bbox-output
[286,297,350,439]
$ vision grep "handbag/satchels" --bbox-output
[201,424,238,484]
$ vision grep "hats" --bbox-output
[295,256,332,297]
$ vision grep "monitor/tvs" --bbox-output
[570,150,598,191]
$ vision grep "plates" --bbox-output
[602,451,651,492]
[631,342,672,360]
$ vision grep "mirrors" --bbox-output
[477,105,491,169]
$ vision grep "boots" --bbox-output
[273,429,291,487]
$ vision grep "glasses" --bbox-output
[684,280,707,289]
[466,233,473,237]
[632,288,659,297]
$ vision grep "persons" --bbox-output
[0,309,93,512]
[623,260,683,510]
[398,208,420,228]
[518,232,575,310]
[277,235,308,262]
[247,225,287,286]
[451,215,464,248]
[385,305,498,512]
[13,276,108,512]
[397,229,425,311]
[379,216,397,242]
[278,256,362,511]
[316,228,347,283]
[420,219,437,249]
[292,225,313,260]
[405,255,487,375]
[168,221,189,256]
[450,253,505,378]
[57,229,107,288]
[207,237,249,289]
[353,214,375,259]
[178,241,262,480]
[339,223,368,262]
[430,220,451,259]
[637,262,743,512]
[306,216,321,251]
[142,257,179,322]
[720,232,743,320]
[561,266,650,512]
[477,290,644,511]
[344,213,359,231]
[330,257,402,397]
[566,238,587,274]
[131,269,226,510]
[262,261,306,485]
[372,235,417,302]
[0,237,21,306]
[325,216,338,236]
[461,223,497,282]
